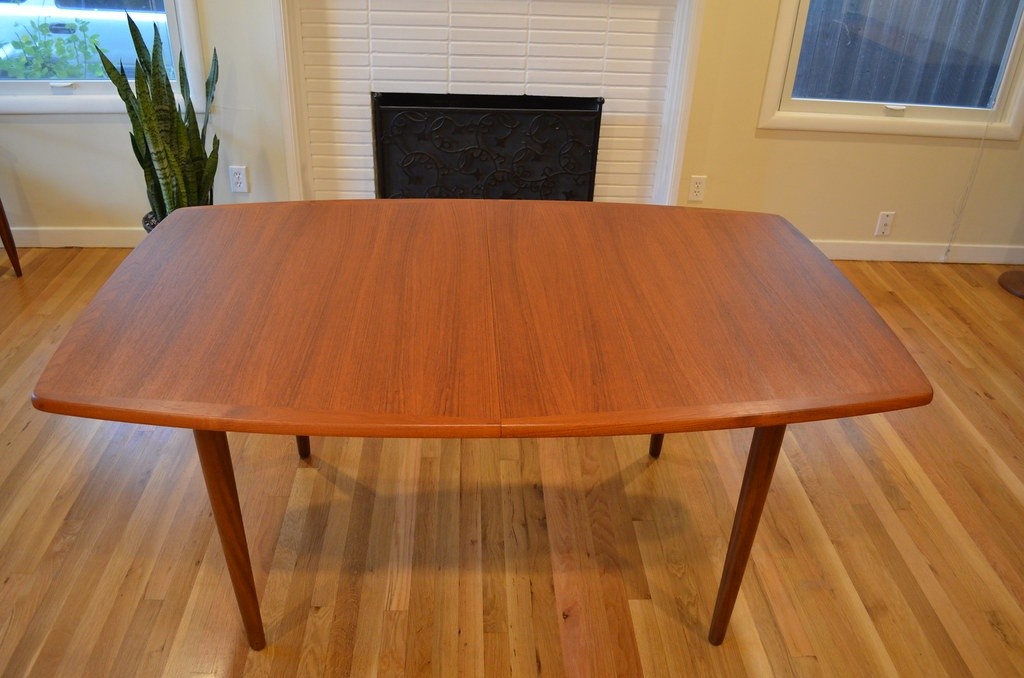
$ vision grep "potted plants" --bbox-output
[86,9,219,231]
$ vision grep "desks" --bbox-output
[16,195,934,650]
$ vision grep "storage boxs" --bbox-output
[0,0,210,115]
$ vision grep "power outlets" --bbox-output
[688,175,707,202]
[229,165,249,193]
[874,212,896,236]
